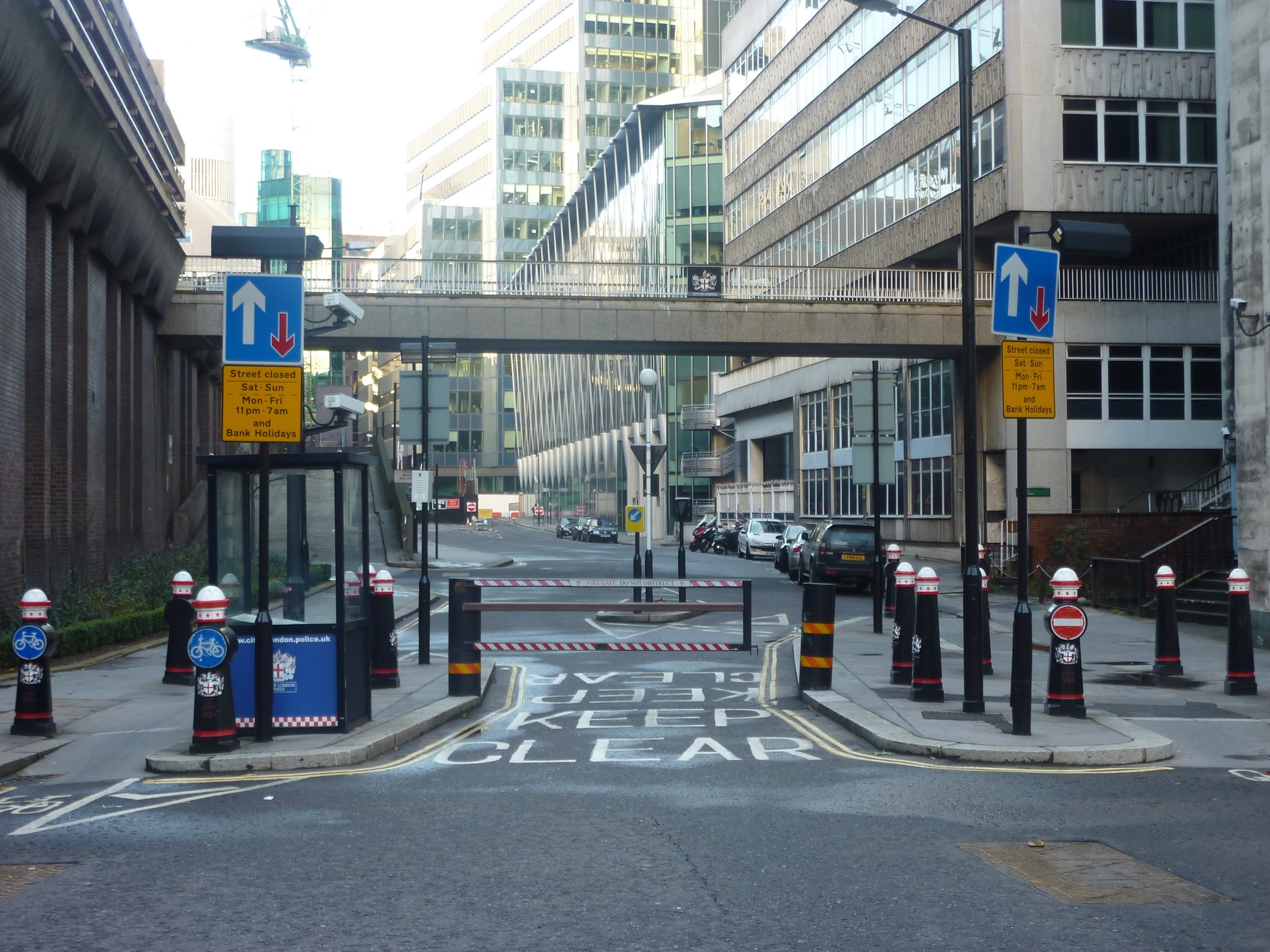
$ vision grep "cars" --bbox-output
[581,517,618,544]
[737,517,787,560]
[786,530,815,581]
[571,516,592,541]
[556,517,578,539]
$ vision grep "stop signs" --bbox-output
[577,507,586,515]
[535,507,543,515]
[467,503,476,511]
[1050,603,1088,641]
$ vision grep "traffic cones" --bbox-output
[466,516,470,526]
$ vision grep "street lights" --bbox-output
[535,454,540,527]
[639,368,659,601]
[282,201,376,621]
[400,336,458,666]
[849,0,985,713]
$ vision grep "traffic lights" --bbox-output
[674,497,693,522]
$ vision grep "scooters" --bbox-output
[689,512,748,555]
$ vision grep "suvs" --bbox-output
[774,522,818,573]
[798,518,887,595]
